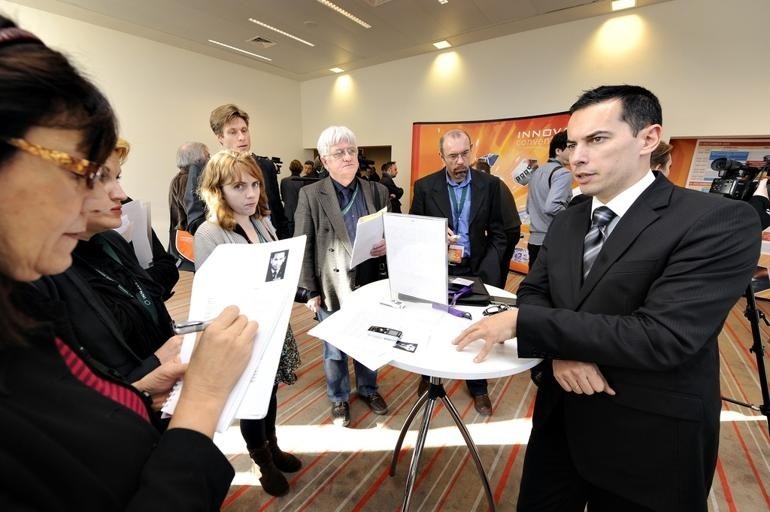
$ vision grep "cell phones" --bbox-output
[368,326,402,341]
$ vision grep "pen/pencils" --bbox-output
[173,321,212,335]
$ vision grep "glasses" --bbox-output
[483,303,508,316]
[8,137,102,190]
[327,147,357,158]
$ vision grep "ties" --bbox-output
[583,205,617,281]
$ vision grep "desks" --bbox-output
[348,274,544,512]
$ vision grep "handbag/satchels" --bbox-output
[168,228,194,273]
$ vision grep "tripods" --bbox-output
[722,280,770,438]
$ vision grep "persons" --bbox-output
[0,15,260,511]
[168,104,524,496]
[452,85,762,512]
[650,141,674,178]
[526,130,572,270]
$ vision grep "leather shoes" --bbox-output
[474,394,492,415]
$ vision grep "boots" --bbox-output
[247,433,302,498]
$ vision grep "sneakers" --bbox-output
[360,391,388,415]
[332,401,351,427]
